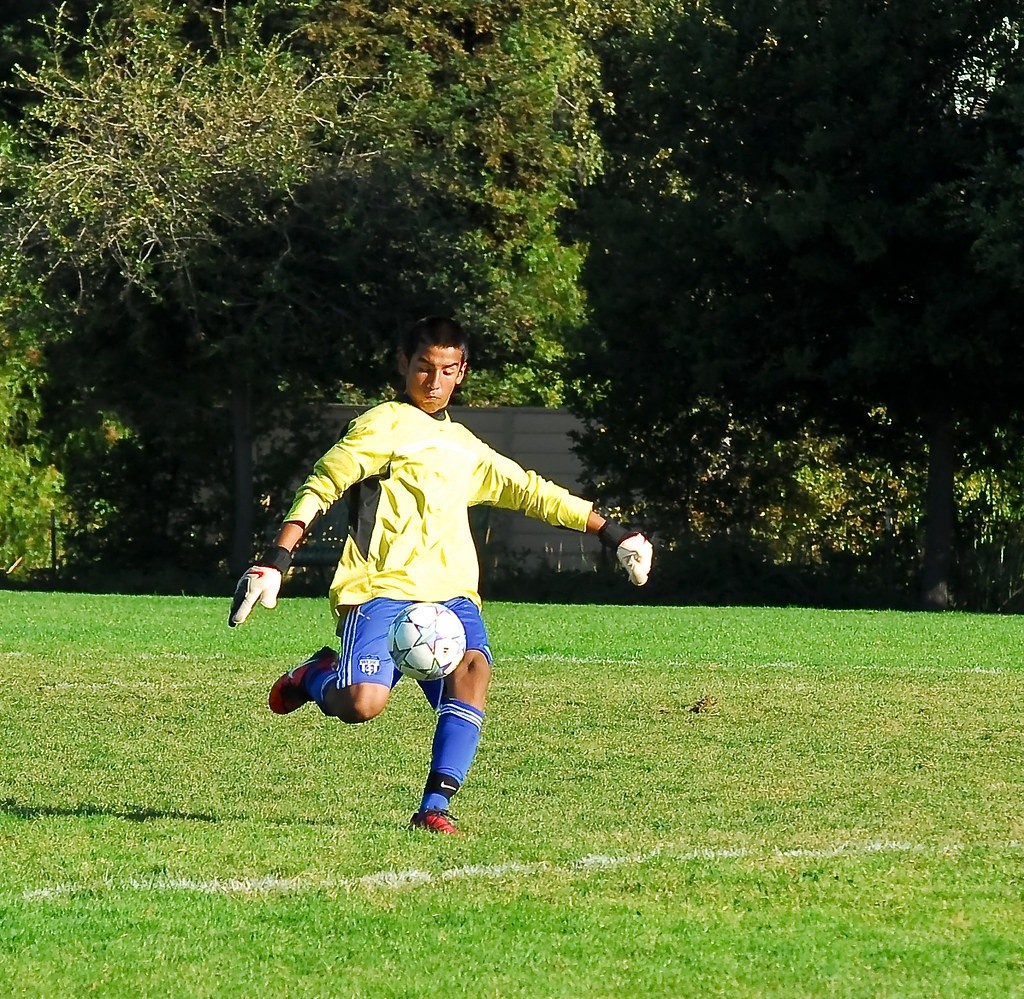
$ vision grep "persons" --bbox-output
[228,315,654,835]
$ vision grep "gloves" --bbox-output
[229,546,293,627]
[600,518,652,587]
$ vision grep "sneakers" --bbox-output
[408,806,461,836]
[270,646,341,714]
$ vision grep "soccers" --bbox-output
[387,602,467,682]
